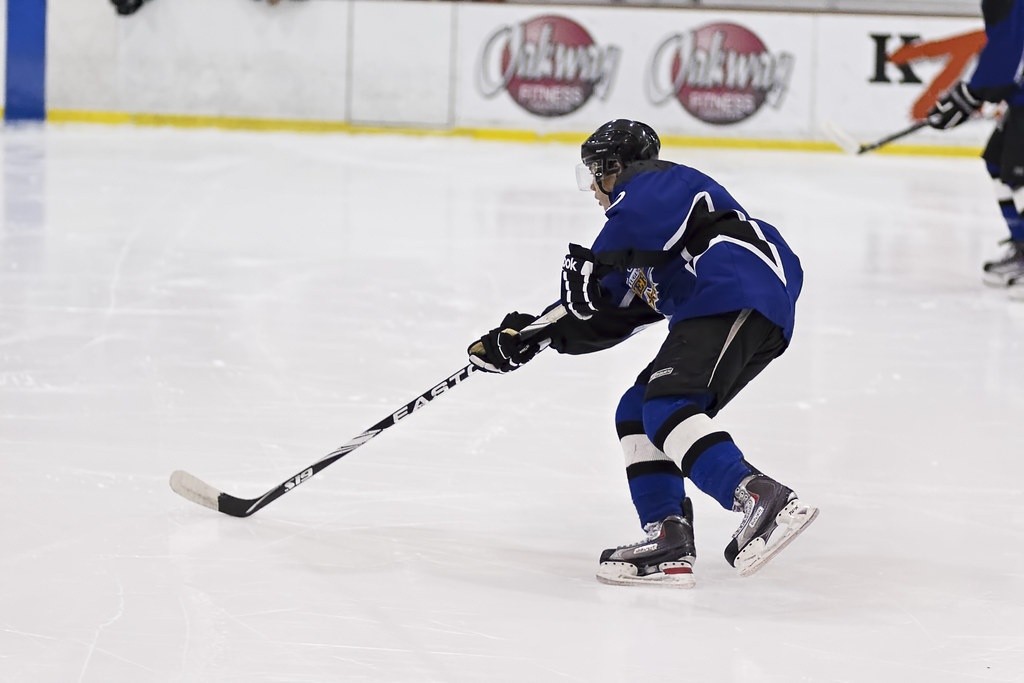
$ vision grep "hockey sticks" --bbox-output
[819,114,943,156]
[166,302,568,519]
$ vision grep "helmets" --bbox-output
[581,119,661,179]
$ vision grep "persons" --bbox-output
[469,120,819,590]
[928,0,1024,302]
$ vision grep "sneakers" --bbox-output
[984,237,1024,286]
[596,496,696,588]
[725,462,819,576]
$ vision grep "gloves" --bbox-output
[468,310,552,374]
[561,243,601,322]
[926,81,984,129]
